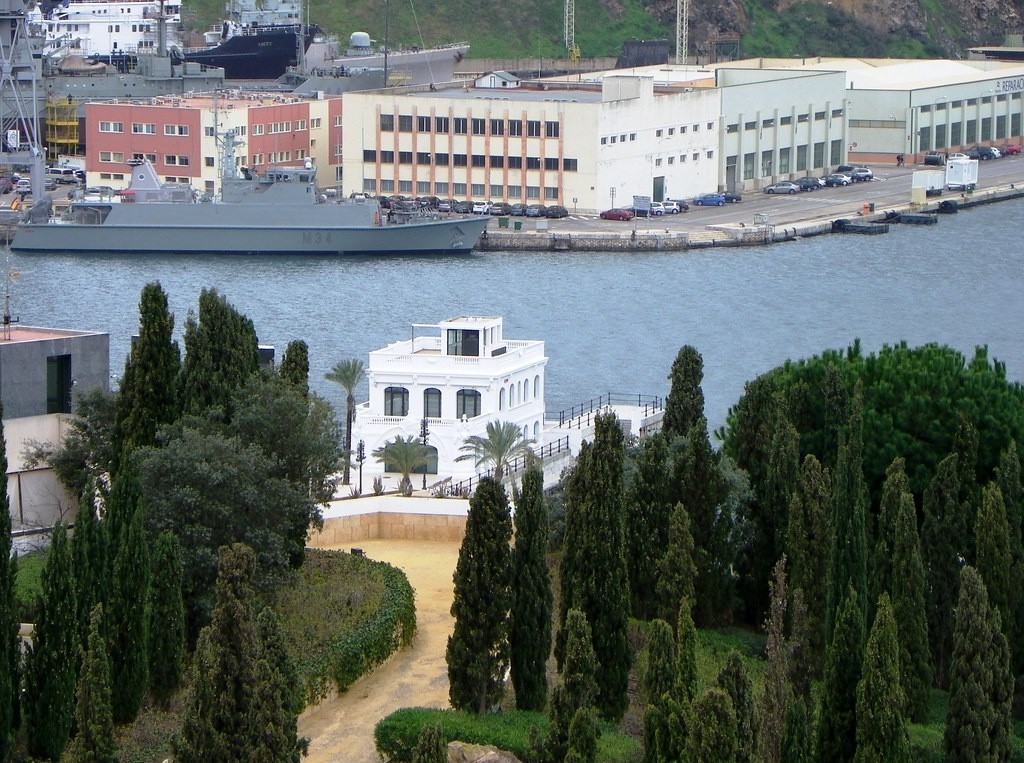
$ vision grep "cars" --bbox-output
[489,203,512,215]
[437,198,458,211]
[600,209,634,221]
[820,176,842,188]
[721,191,742,204]
[800,176,819,190]
[625,202,665,216]
[472,201,493,213]
[526,204,546,217]
[669,200,689,213]
[851,167,873,182]
[847,172,863,182]
[693,193,725,206]
[795,179,813,191]
[455,201,473,213]
[0,164,85,194]
[86,186,113,192]
[831,174,852,185]
[511,204,529,216]
[662,201,680,214]
[818,178,827,188]
[349,192,441,210]
[763,182,801,194]
[545,205,568,219]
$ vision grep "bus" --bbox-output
[46,168,73,185]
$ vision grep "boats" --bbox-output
[9,90,490,252]
[22,0,319,80]
[297,36,471,85]
[313,316,668,498]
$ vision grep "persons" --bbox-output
[66,93,74,104]
[896,155,906,167]
